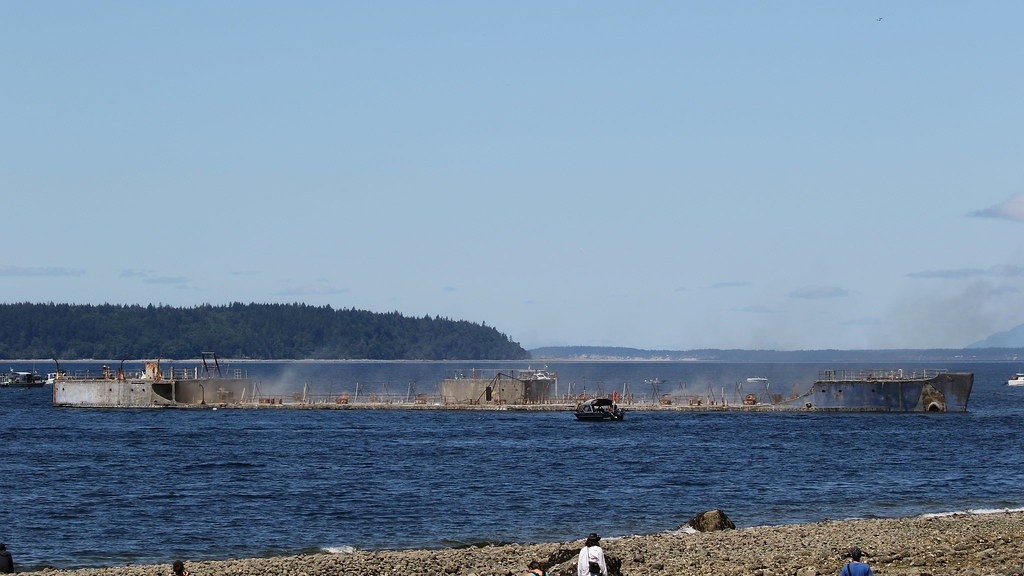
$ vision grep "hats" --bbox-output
[588,533,601,540]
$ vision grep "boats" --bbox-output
[573,390,627,422]
[516,364,559,384]
[0,357,70,388]
[1007,372,1024,387]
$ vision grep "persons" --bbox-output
[578,532,608,576]
[524,561,548,576]
[839,546,875,576]
[168,560,190,576]
[0,543,15,574]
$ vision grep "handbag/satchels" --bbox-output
[589,561,599,573]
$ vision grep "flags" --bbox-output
[613,391,618,401]
